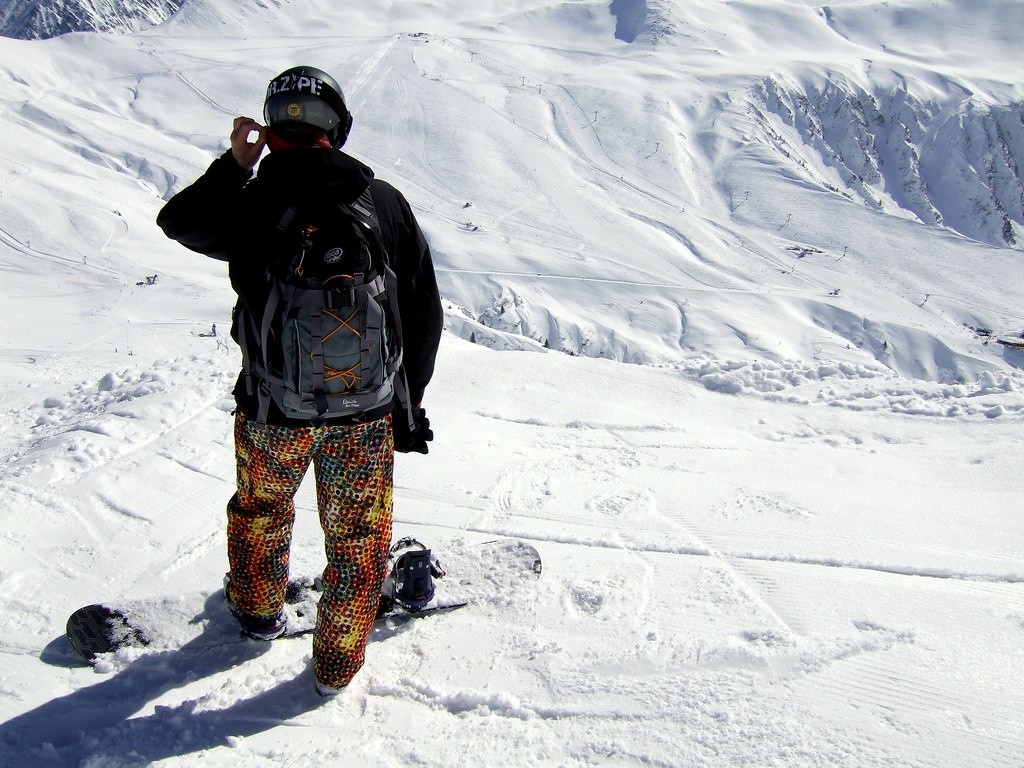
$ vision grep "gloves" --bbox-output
[394,407,434,455]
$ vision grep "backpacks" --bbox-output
[244,179,404,420]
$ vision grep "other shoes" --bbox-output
[224,571,288,640]
[314,674,348,697]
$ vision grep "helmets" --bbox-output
[264,66,353,150]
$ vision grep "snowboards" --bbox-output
[67,539,542,663]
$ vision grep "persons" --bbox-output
[155,66,443,698]
